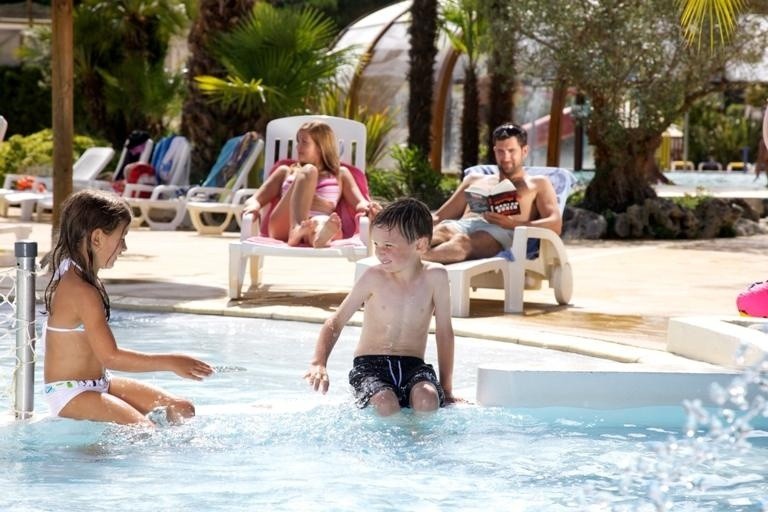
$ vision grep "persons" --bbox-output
[240,120,382,246]
[41,189,214,431]
[371,120,562,262]
[300,200,467,415]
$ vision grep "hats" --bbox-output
[124,129,150,147]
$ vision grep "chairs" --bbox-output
[0,134,267,236]
[221,111,374,306]
[358,161,579,320]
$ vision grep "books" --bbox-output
[463,178,521,218]
